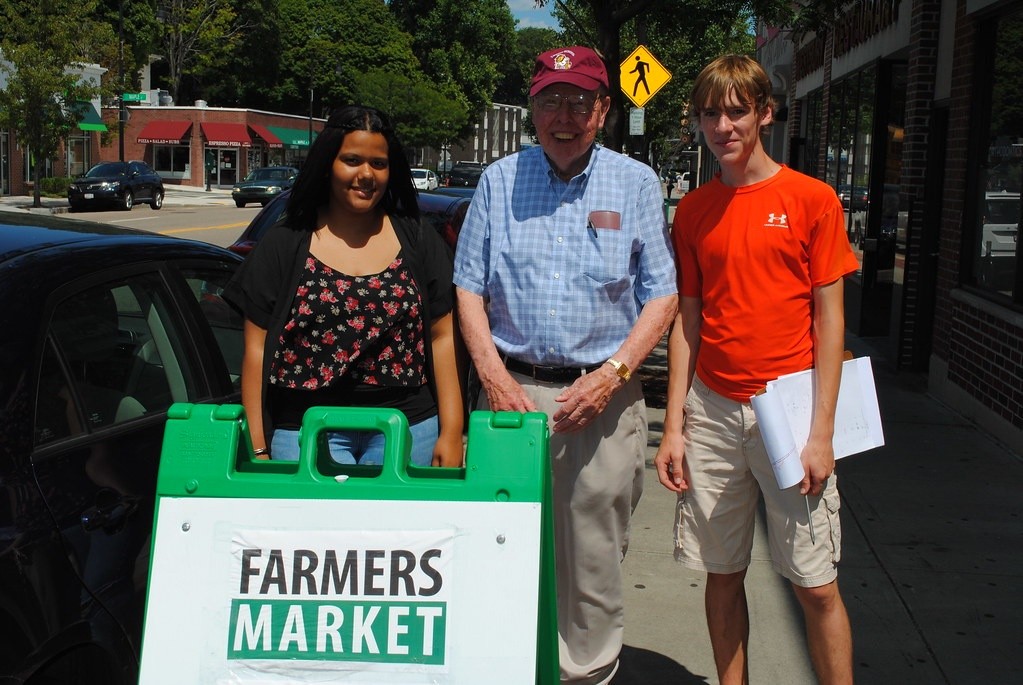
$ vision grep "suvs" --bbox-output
[982,190,1022,269]
[67,160,166,211]
[439,160,491,188]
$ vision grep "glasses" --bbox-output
[534,93,602,114]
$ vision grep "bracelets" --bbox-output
[254,448,269,454]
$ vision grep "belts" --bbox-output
[498,352,603,384]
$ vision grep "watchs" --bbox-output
[607,357,631,382]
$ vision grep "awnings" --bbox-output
[200,122,252,148]
[137,120,193,144]
[248,123,319,151]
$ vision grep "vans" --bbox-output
[681,172,692,196]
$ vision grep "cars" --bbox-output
[838,184,870,209]
[227,186,479,260]
[0,208,246,683]
[898,211,908,242]
[230,166,300,208]
[410,167,437,192]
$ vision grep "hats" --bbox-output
[529,46,609,97]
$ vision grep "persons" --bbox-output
[666,175,675,198]
[222,106,464,468]
[453,45,678,685]
[654,53,860,685]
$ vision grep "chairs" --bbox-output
[56,290,148,428]
